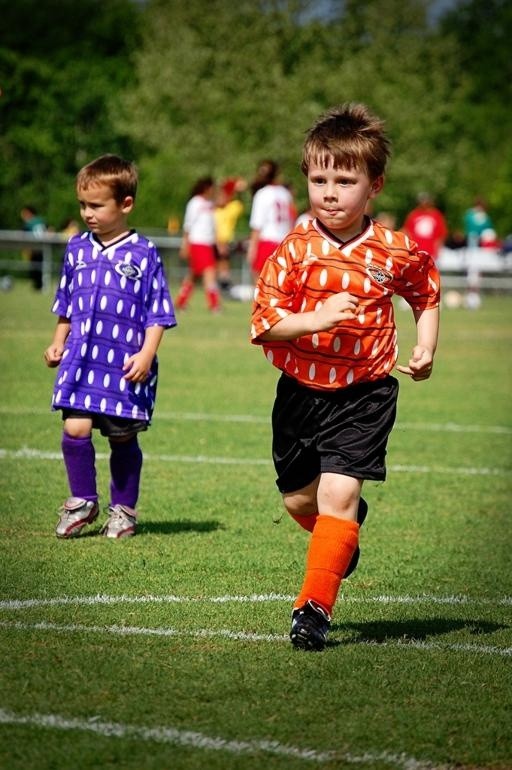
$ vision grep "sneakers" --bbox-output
[98,503,138,538]
[342,496,368,578]
[290,600,332,651]
[54,497,99,538]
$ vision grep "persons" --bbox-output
[396,190,449,311]
[248,102,443,653]
[58,216,82,236]
[213,178,247,302]
[249,158,297,298]
[41,154,178,541]
[273,174,299,221]
[478,228,501,295]
[463,198,497,305]
[171,175,227,317]
[20,204,49,291]
